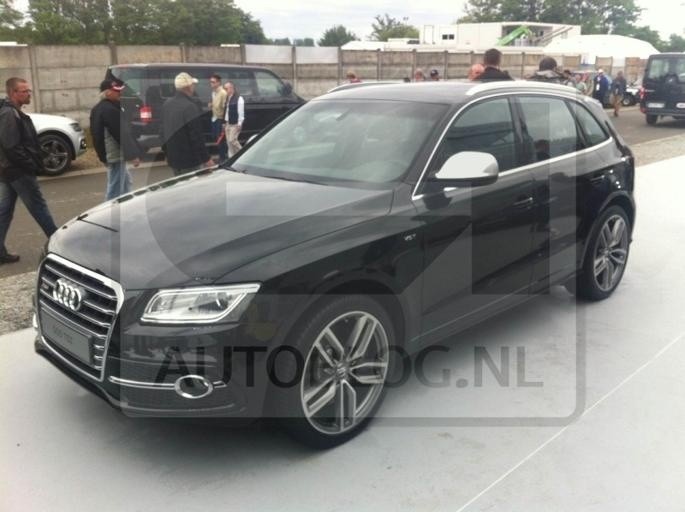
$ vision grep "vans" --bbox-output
[639,52,685,126]
[99,63,311,162]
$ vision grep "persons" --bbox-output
[276,76,293,98]
[533,138,568,231]
[346,48,627,118]
[220,81,246,159]
[90,78,143,202]
[1,77,57,262]
[158,72,213,177]
[207,74,225,164]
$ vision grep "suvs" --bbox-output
[32,79,636,449]
[572,69,640,106]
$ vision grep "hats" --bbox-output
[100,78,126,92]
[175,71,199,89]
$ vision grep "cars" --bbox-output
[19,113,86,173]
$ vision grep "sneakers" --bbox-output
[0,254,19,265]
